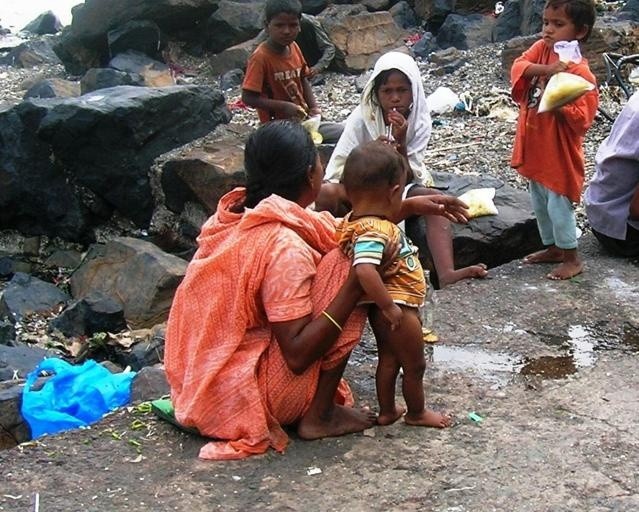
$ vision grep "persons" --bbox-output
[332,139,452,428]
[510,0,599,281]
[315,51,488,289]
[241,1,345,144]
[164,118,472,460]
[584,90,639,258]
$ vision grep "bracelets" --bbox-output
[321,310,344,332]
